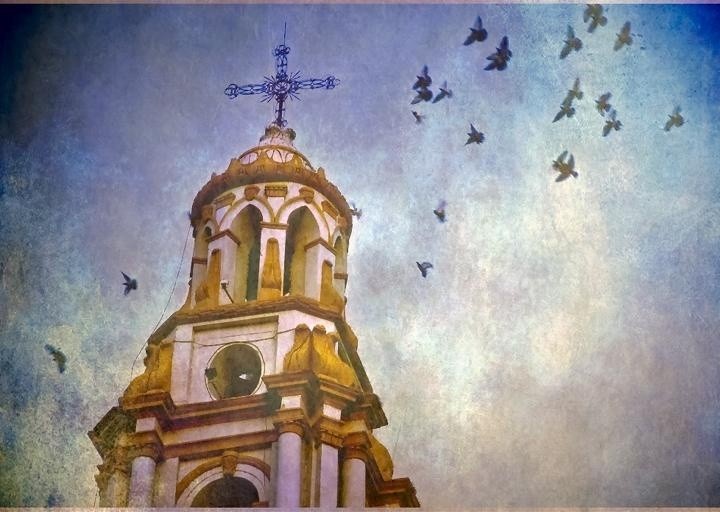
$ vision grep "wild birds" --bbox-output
[432,205,447,224]
[410,64,453,126]
[462,16,488,47]
[44,343,67,374]
[416,261,434,278]
[663,106,685,133]
[483,36,514,72]
[548,2,633,138]
[464,123,485,147]
[121,272,137,297]
[551,150,578,183]
